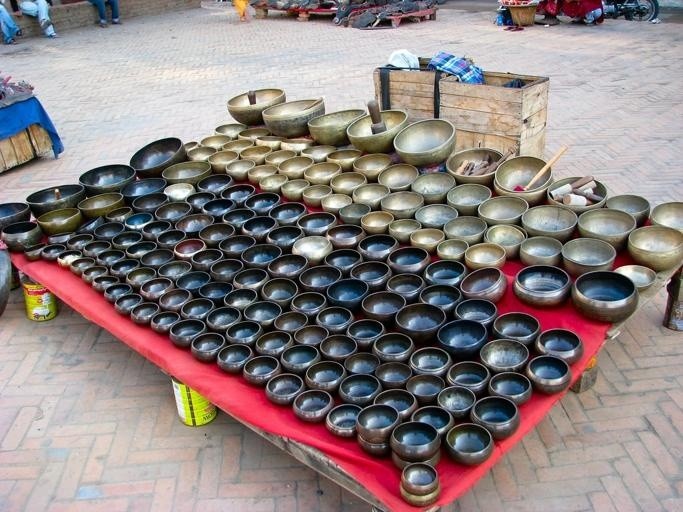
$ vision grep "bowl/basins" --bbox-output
[316,306,353,335]
[163,182,197,201]
[307,109,366,147]
[445,422,493,465]
[120,179,166,197]
[263,150,297,166]
[346,109,409,154]
[126,267,157,288]
[325,223,365,248]
[267,254,310,280]
[158,288,192,312]
[405,374,445,402]
[123,213,154,231]
[296,211,336,235]
[75,214,103,234]
[130,137,186,178]
[373,389,417,420]
[495,155,551,192]
[222,139,254,154]
[290,292,328,319]
[103,282,133,303]
[571,270,639,324]
[355,402,399,443]
[346,319,386,347]
[484,223,525,257]
[302,184,332,207]
[184,141,198,151]
[356,434,389,457]
[114,293,143,313]
[399,484,440,507]
[325,149,362,170]
[205,307,242,332]
[156,229,187,248]
[400,462,439,495]
[191,249,224,272]
[390,421,441,461]
[478,194,529,223]
[237,127,271,142]
[254,330,294,357]
[232,268,269,289]
[264,373,305,405]
[158,260,192,279]
[280,139,315,153]
[464,243,507,269]
[280,344,320,372]
[201,135,232,151]
[201,198,235,219]
[436,238,469,261]
[218,235,257,258]
[513,263,571,306]
[92,221,125,240]
[577,207,636,252]
[226,320,263,345]
[256,135,282,149]
[291,235,332,265]
[207,150,239,173]
[392,119,457,168]
[131,301,159,322]
[488,372,531,406]
[126,241,157,258]
[214,123,247,139]
[266,202,306,224]
[259,174,288,192]
[47,232,73,243]
[459,266,508,301]
[1,220,42,252]
[281,179,310,201]
[343,351,380,374]
[25,183,84,213]
[546,176,608,212]
[195,173,233,195]
[210,258,245,280]
[325,404,362,437]
[319,335,358,361]
[141,221,172,240]
[361,291,406,322]
[161,161,212,185]
[357,234,400,261]
[77,192,121,218]
[422,260,468,287]
[180,298,215,320]
[226,88,285,124]
[220,184,255,206]
[320,194,352,212]
[199,281,234,299]
[494,311,540,342]
[248,164,277,184]
[240,244,282,268]
[338,373,381,402]
[105,206,133,223]
[96,251,126,265]
[111,231,143,249]
[614,264,656,293]
[0,202,30,228]
[535,328,583,364]
[278,156,314,180]
[303,162,342,184]
[323,248,363,273]
[262,98,325,138]
[526,353,571,394]
[454,298,499,327]
[409,346,452,375]
[385,273,425,301]
[350,261,393,288]
[409,228,445,252]
[380,191,425,219]
[83,240,111,257]
[326,279,370,310]
[338,203,371,224]
[480,337,530,373]
[242,355,281,386]
[241,216,277,240]
[351,153,388,178]
[360,211,393,234]
[414,203,458,227]
[411,171,456,203]
[243,300,282,325]
[40,244,67,259]
[650,202,682,232]
[298,266,342,292]
[176,272,211,291]
[274,311,308,334]
[445,183,492,215]
[469,395,520,441]
[140,248,174,269]
[411,405,455,437]
[154,201,193,223]
[132,193,170,213]
[605,194,650,225]
[56,250,82,265]
[191,333,226,363]
[388,219,422,243]
[150,311,181,333]
[294,325,329,347]
[445,360,490,396]
[174,213,215,234]
[437,319,488,356]
[446,147,506,183]
[110,259,140,278]
[186,146,217,160]
[243,191,280,214]
[216,344,254,373]
[352,183,390,207]
[225,160,255,182]
[391,449,441,473]
[304,360,348,392]
[265,226,305,250]
[173,238,207,261]
[185,191,215,209]
[198,222,236,244]
[443,215,487,245]
[69,257,96,273]
[66,234,94,249]
[627,225,682,269]
[139,277,174,299]
[300,145,336,163]
[436,386,476,418]
[221,208,257,228]
[169,318,207,346]
[519,204,577,242]
[223,288,258,310]
[375,361,412,388]
[419,283,463,310]
[261,278,298,307]
[394,303,447,343]
[486,225,525,244]
[92,274,120,291]
[518,236,561,265]
[79,163,136,192]
[82,265,108,283]
[292,389,334,423]
[23,243,45,259]
[36,207,82,234]
[491,180,551,204]
[240,145,272,165]
[329,172,368,195]
[386,246,430,275]
[372,332,413,363]
[377,163,419,192]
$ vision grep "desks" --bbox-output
[0,97,53,174]
[250,0,439,28]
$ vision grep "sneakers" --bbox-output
[17,29,23,36]
[40,19,50,30]
[111,19,121,23]
[99,20,108,28]
[9,39,17,44]
[49,33,64,39]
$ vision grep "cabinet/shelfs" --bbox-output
[1,123,683,512]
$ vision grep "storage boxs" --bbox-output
[439,72,550,158]
[374,59,442,122]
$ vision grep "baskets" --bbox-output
[503,4,537,26]
